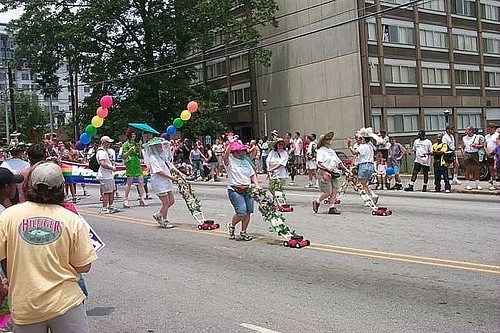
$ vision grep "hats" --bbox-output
[225,140,250,150]
[142,137,172,166]
[0,167,24,184]
[317,132,334,149]
[101,136,114,143]
[31,163,65,189]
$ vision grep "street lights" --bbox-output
[261,100,269,143]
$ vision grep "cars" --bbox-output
[283,235,310,249]
[278,204,293,212]
[262,204,278,212]
[324,197,341,204]
[371,207,392,216]
[198,220,220,230]
[449,145,490,181]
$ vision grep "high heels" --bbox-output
[123,202,130,208]
[140,200,149,207]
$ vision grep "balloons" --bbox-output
[75,95,112,150]
[385,167,394,175]
[160,100,199,141]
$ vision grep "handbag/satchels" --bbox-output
[443,149,455,164]
[231,185,249,194]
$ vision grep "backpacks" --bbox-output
[89,148,104,172]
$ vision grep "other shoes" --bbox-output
[372,194,379,207]
[329,207,341,214]
[313,200,320,213]
[404,184,415,191]
[391,182,403,190]
[153,211,174,228]
[304,182,319,187]
[109,205,121,212]
[422,185,428,192]
[240,230,252,241]
[73,191,90,204]
[451,179,497,190]
[100,207,114,214]
[226,222,235,239]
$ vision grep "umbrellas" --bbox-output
[128,123,161,134]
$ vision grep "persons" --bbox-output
[0,123,500,333]
